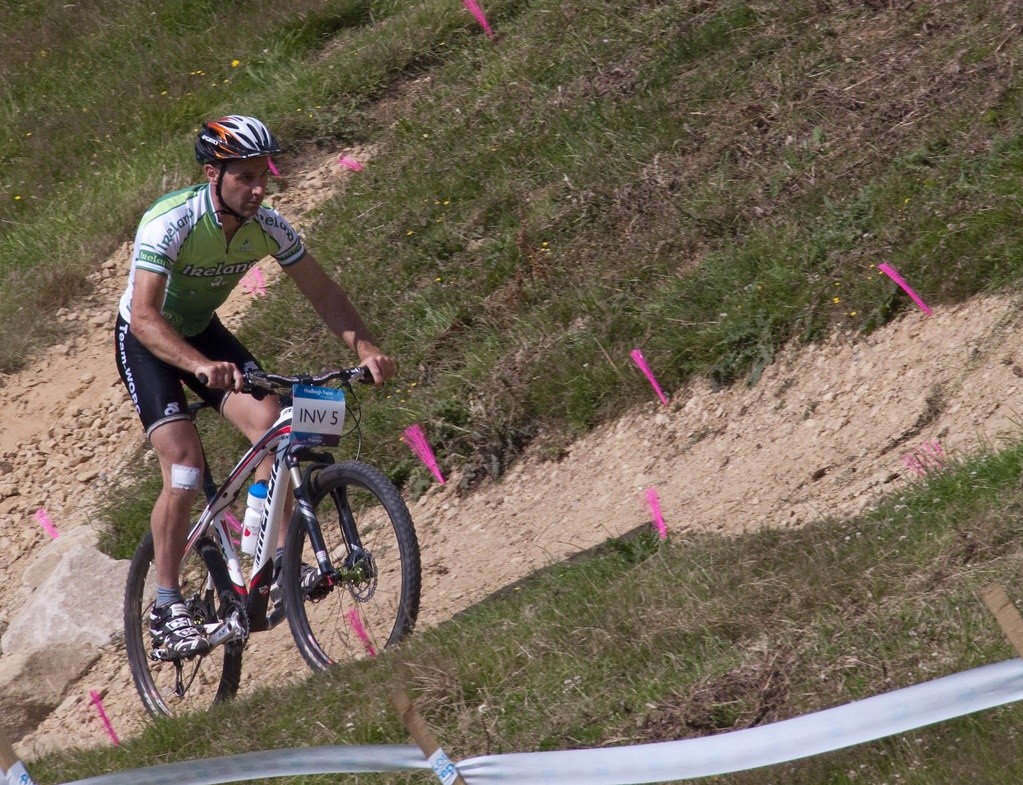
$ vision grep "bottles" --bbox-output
[241,479,268,556]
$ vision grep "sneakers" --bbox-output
[148,601,212,661]
[269,563,342,604]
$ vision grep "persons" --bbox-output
[115,115,396,661]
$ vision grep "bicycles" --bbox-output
[122,372,423,726]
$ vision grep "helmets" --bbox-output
[195,115,282,165]
